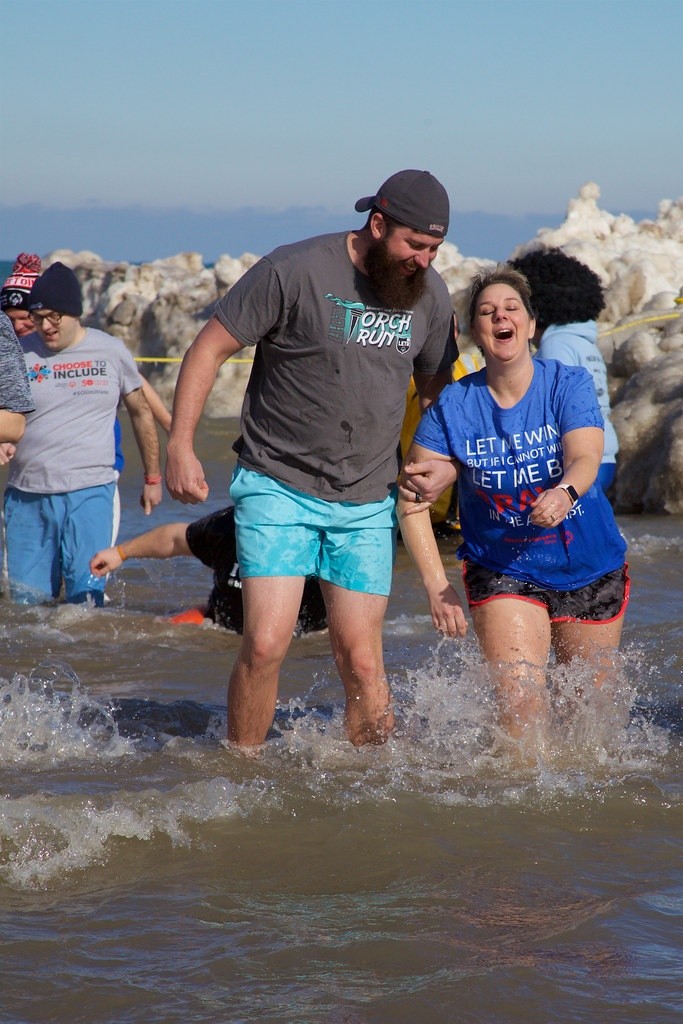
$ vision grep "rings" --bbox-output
[415,491,420,503]
[551,514,557,522]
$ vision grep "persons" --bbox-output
[395,306,487,537]
[89,502,331,640]
[0,310,38,446]
[395,261,633,774]
[1,260,164,609]
[0,252,163,605]
[504,245,622,501]
[163,167,460,762]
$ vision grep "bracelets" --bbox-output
[118,544,128,562]
[143,473,163,486]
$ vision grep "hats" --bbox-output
[27,262,82,316]
[0,253,41,312]
[355,170,450,236]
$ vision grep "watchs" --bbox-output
[554,483,579,510]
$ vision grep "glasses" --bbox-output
[27,311,65,324]
